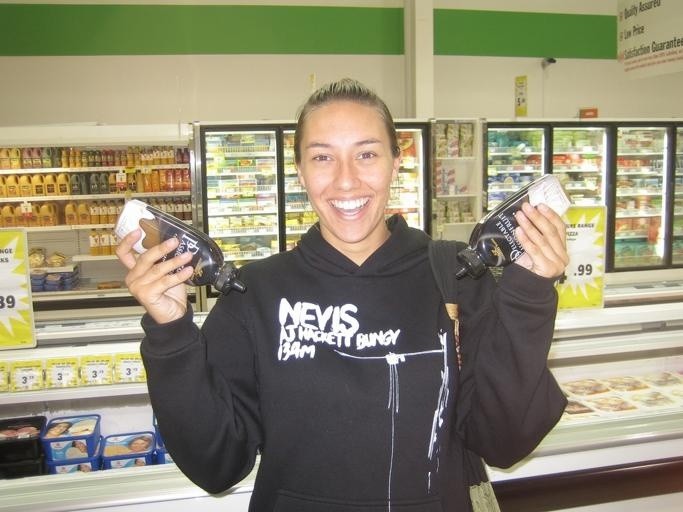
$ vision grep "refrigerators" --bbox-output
[484,118,683,298]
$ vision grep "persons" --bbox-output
[114,78,568,512]
[44,421,152,472]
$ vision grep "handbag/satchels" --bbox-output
[428,237,501,512]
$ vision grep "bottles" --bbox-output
[0,149,193,256]
[115,200,247,302]
[455,174,568,280]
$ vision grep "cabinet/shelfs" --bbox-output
[433,115,483,249]
[0,323,260,512]
[0,117,200,311]
[483,115,683,272]
[470,300,682,512]
